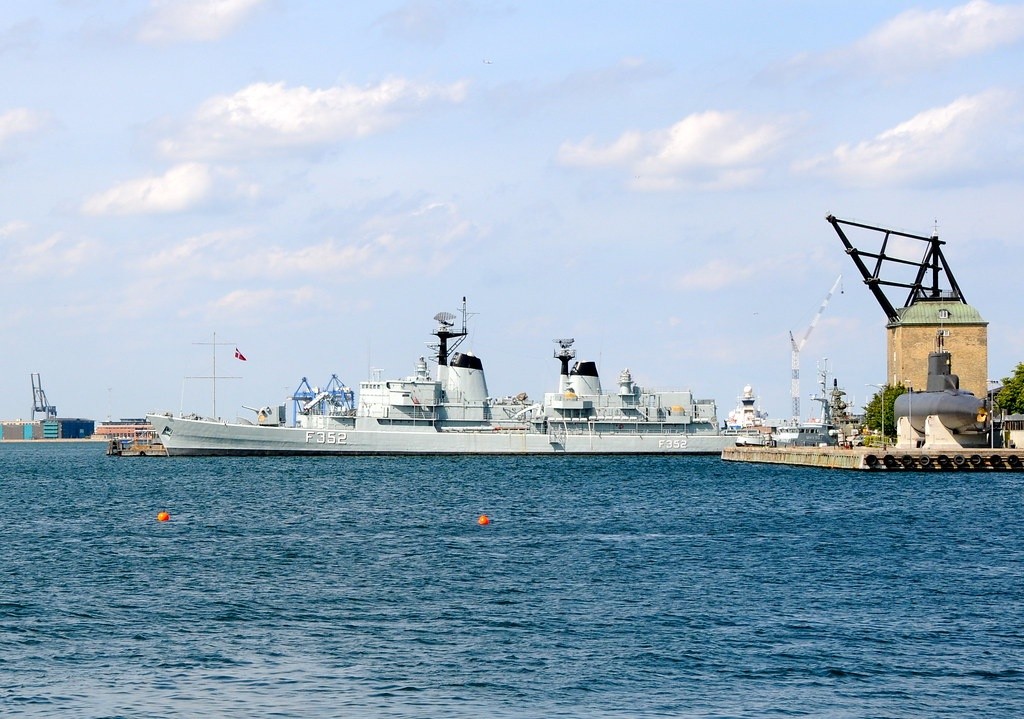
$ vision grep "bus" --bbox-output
[771,423,837,446]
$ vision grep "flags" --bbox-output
[235,348,246,361]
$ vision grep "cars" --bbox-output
[852,435,867,446]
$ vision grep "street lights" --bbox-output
[985,380,999,449]
[905,380,912,449]
[864,384,887,448]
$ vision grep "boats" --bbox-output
[146,295,777,456]
[105,435,167,457]
[735,433,765,447]
[721,329,1024,472]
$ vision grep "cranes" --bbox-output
[788,269,841,426]
